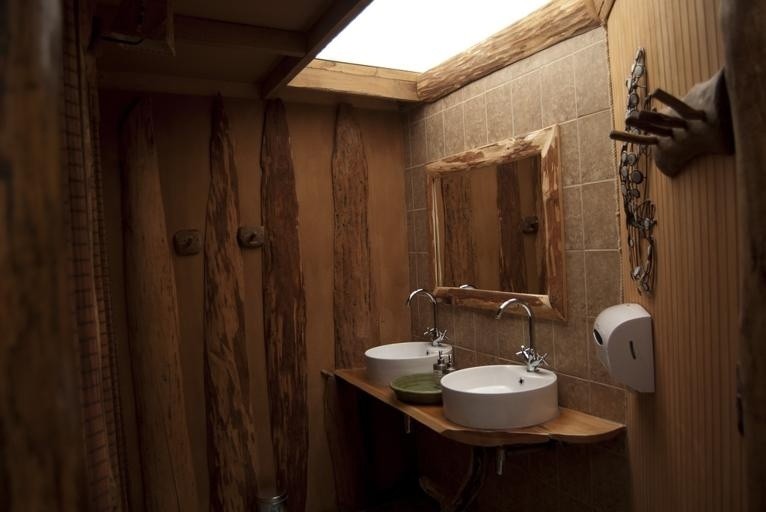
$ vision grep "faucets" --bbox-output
[460,284,478,290]
[495,298,537,361]
[405,288,439,337]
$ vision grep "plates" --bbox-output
[389,374,451,406]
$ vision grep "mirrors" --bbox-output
[423,122,570,324]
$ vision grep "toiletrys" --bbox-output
[444,353,455,374]
[433,349,447,385]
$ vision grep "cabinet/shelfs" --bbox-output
[332,369,627,511]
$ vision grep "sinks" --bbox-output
[365,340,454,387]
[439,364,560,431]
